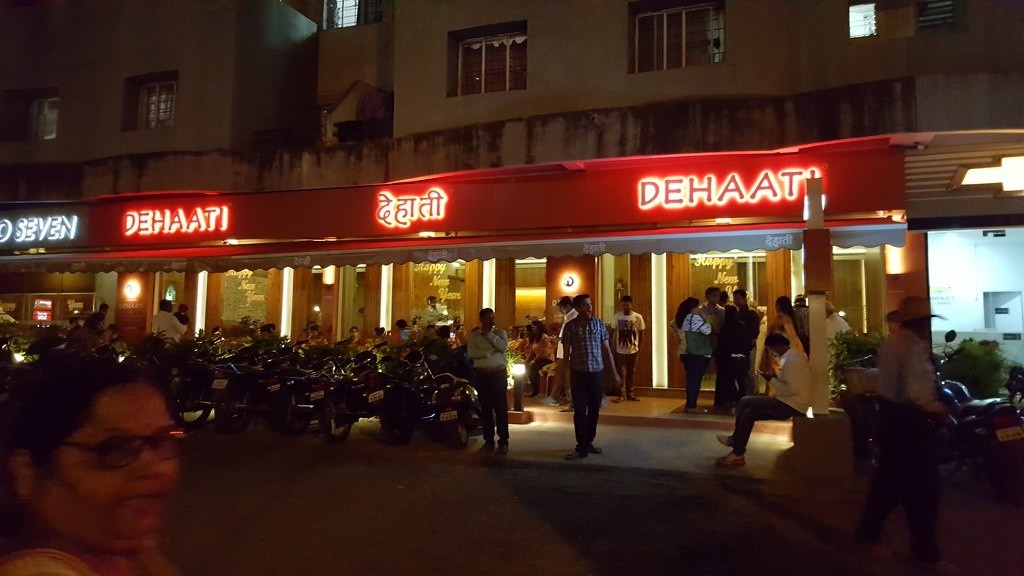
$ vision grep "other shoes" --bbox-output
[565,449,589,460]
[529,391,541,399]
[851,541,894,562]
[627,394,641,400]
[585,443,602,454]
[916,560,958,576]
[496,442,509,454]
[479,439,494,452]
[612,396,626,403]
[685,404,697,413]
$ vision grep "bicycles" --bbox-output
[831,354,882,466]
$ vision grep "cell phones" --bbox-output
[757,369,763,374]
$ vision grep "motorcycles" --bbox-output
[929,328,1024,508]
[0,316,487,449]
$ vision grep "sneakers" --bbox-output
[716,451,746,465]
[716,434,736,447]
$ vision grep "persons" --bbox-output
[856,296,955,572]
[668,286,767,416]
[174,305,190,325]
[367,296,465,350]
[760,296,808,396]
[299,322,327,347]
[611,296,645,402]
[153,300,188,347]
[68,304,129,359]
[825,301,850,378]
[0,356,179,576]
[208,324,275,350]
[343,327,367,348]
[793,293,810,352]
[716,333,811,467]
[561,295,621,458]
[467,309,509,455]
[507,297,577,412]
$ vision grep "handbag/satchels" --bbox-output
[684,315,713,356]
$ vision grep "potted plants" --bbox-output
[828,329,889,459]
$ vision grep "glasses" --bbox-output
[56,425,189,473]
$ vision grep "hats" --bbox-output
[886,295,931,323]
